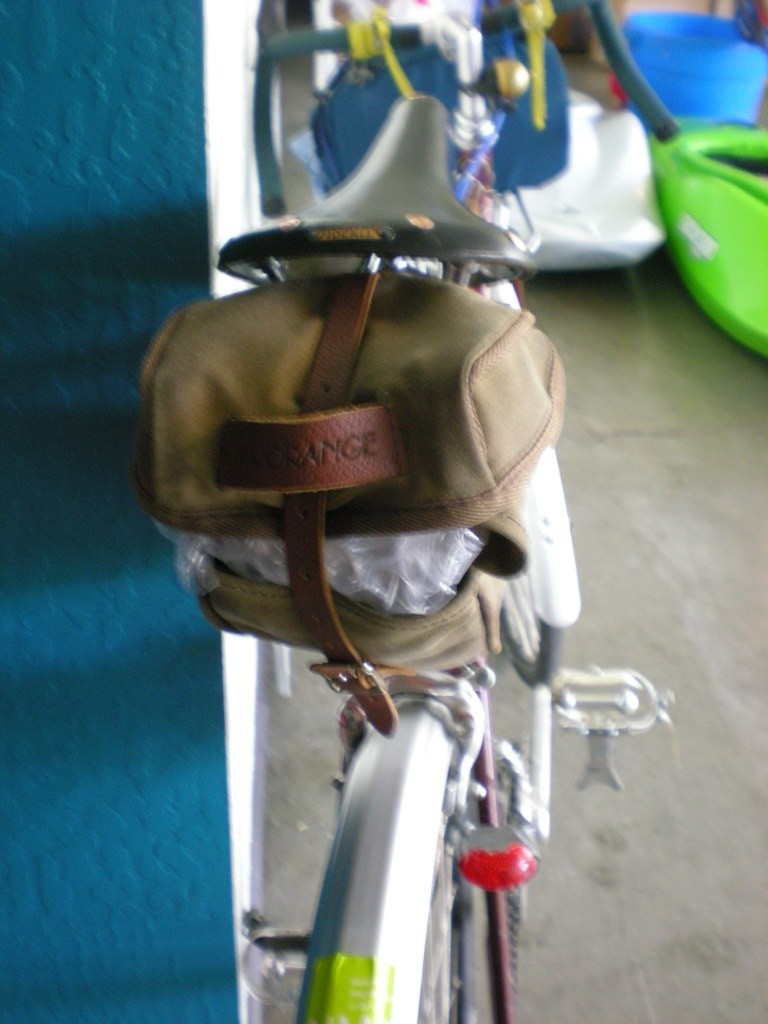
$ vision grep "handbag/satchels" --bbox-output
[309,0,569,193]
[129,273,566,738]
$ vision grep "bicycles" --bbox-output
[216,0,669,1024]
